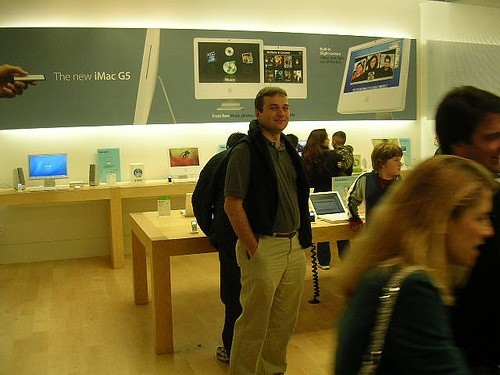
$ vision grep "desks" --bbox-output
[129,208,368,356]
[0,177,198,270]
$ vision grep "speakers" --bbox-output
[13,168,26,190]
[89,164,99,185]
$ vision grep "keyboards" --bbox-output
[29,186,70,192]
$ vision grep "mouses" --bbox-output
[74,185,81,188]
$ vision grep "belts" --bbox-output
[268,230,300,239]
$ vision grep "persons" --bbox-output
[330,155,496,375]
[432,86,500,375]
[351,55,393,83]
[0,65,38,98]
[346,143,403,234]
[286,128,353,270]
[224,87,312,375]
[191,133,249,364]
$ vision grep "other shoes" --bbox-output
[216,347,231,362]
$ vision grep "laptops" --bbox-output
[309,191,349,222]
[180,193,194,217]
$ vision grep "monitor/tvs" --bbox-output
[165,146,201,179]
[27,153,69,186]
[337,38,411,115]
[193,38,308,110]
